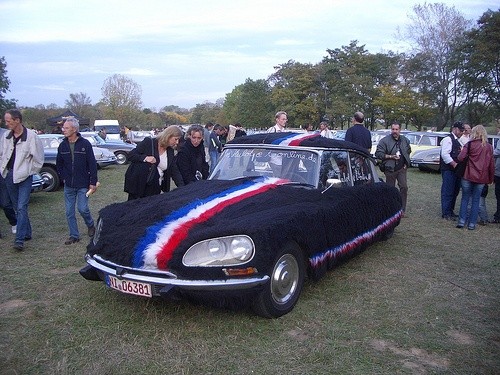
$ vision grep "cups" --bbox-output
[395,151,401,158]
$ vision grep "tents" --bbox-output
[47,110,90,132]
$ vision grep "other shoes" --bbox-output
[451,213,458,217]
[482,220,487,225]
[443,216,456,221]
[13,242,23,251]
[88,226,95,236]
[25,237,32,241]
[488,216,500,223]
[468,222,475,229]
[65,237,78,245]
[456,223,464,228]
[12,225,17,233]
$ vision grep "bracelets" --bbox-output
[390,155,391,158]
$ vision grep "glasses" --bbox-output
[278,118,288,121]
[62,126,75,132]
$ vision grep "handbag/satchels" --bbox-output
[379,163,384,172]
[455,162,465,177]
[124,163,156,196]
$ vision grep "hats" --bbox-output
[453,121,465,130]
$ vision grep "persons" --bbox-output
[0,115,17,234]
[125,125,184,201]
[265,112,288,178]
[320,122,332,138]
[56,117,97,245]
[375,122,411,213]
[98,128,106,140]
[456,124,500,230]
[303,123,313,130]
[178,124,209,184]
[440,122,466,219]
[0,110,44,251]
[204,122,247,172]
[427,127,435,132]
[31,126,38,134]
[345,112,372,179]
[120,125,134,144]
[151,128,158,136]
[81,124,92,131]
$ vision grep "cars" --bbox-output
[78,127,405,319]
[29,120,500,193]
